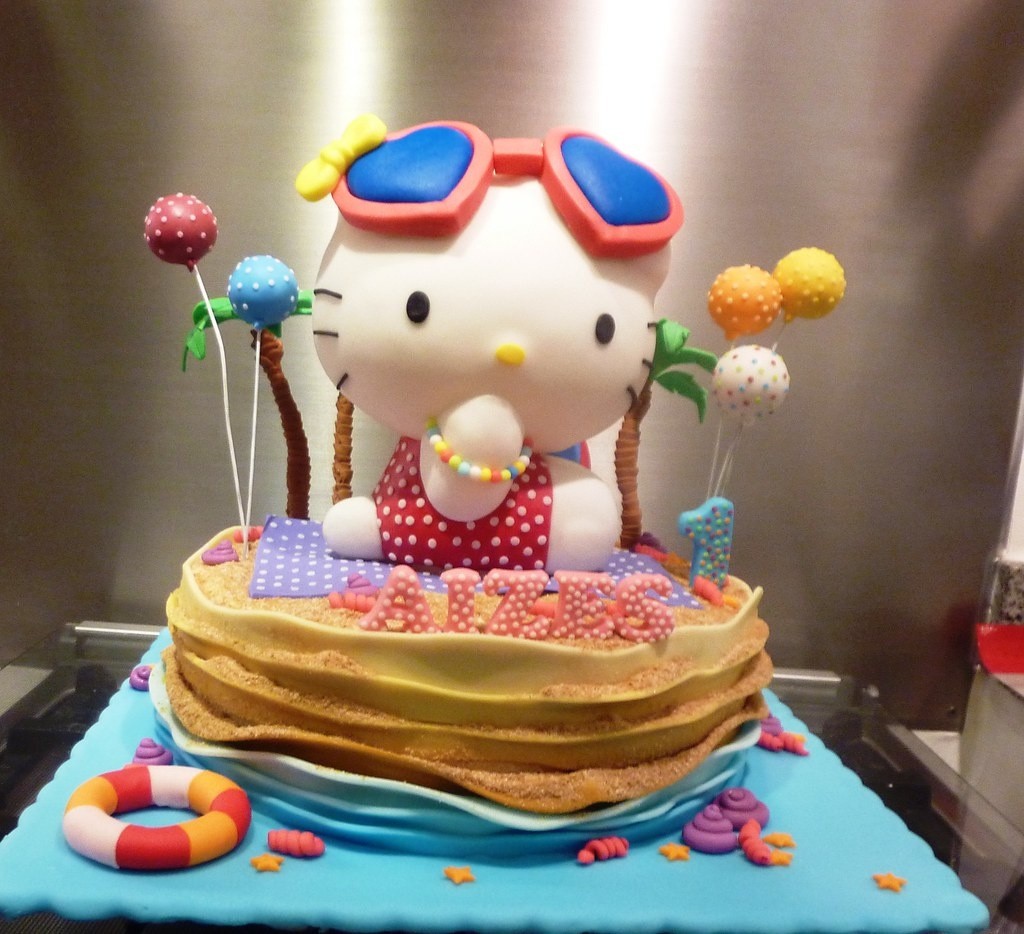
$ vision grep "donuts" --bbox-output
[62,764,250,874]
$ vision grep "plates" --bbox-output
[0,618,989,930]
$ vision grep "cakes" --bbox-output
[149,112,845,859]
